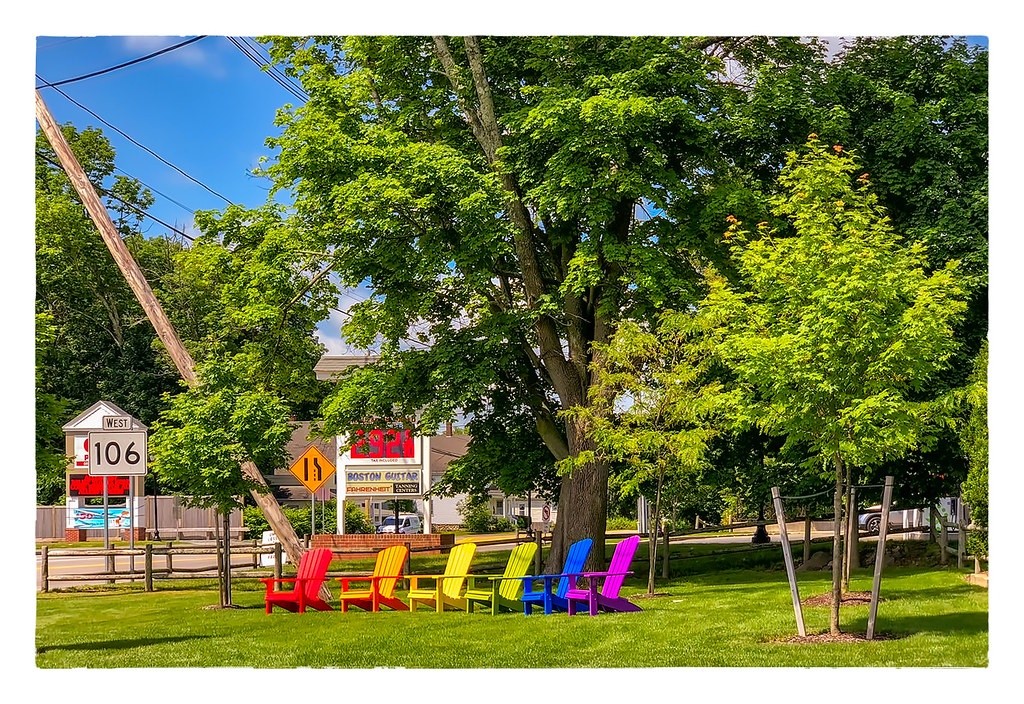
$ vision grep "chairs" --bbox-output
[404,543,480,613]
[519,539,593,616]
[260,547,334,614]
[334,546,410,612]
[464,542,538,616]
[561,534,642,617]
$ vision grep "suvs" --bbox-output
[377,511,423,534]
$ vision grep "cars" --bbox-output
[857,497,930,533]
